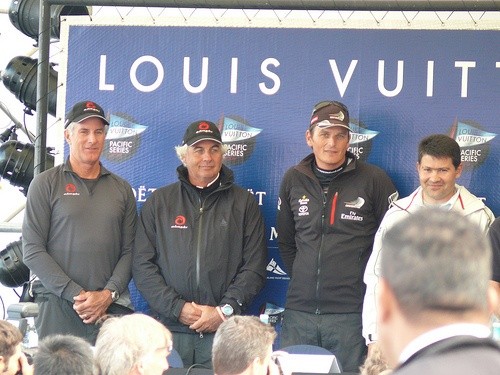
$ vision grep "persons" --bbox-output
[487,217,500,341]
[0,320,32,375]
[361,135,497,347]
[359,343,391,375]
[131,120,270,369]
[32,334,97,375]
[211,315,278,375]
[22,101,138,346]
[276,101,400,372]
[92,311,174,375]
[374,206,500,375]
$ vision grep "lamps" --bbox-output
[0,125,54,195]
[9,0,90,40]
[2,57,59,116]
[0,240,30,288]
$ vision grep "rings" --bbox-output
[84,314,87,319]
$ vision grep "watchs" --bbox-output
[106,288,119,302]
[220,303,235,320]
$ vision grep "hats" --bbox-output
[65,101,110,129]
[310,101,353,135]
[183,120,224,147]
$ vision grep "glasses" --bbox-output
[312,101,349,114]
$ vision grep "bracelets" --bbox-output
[216,306,226,323]
